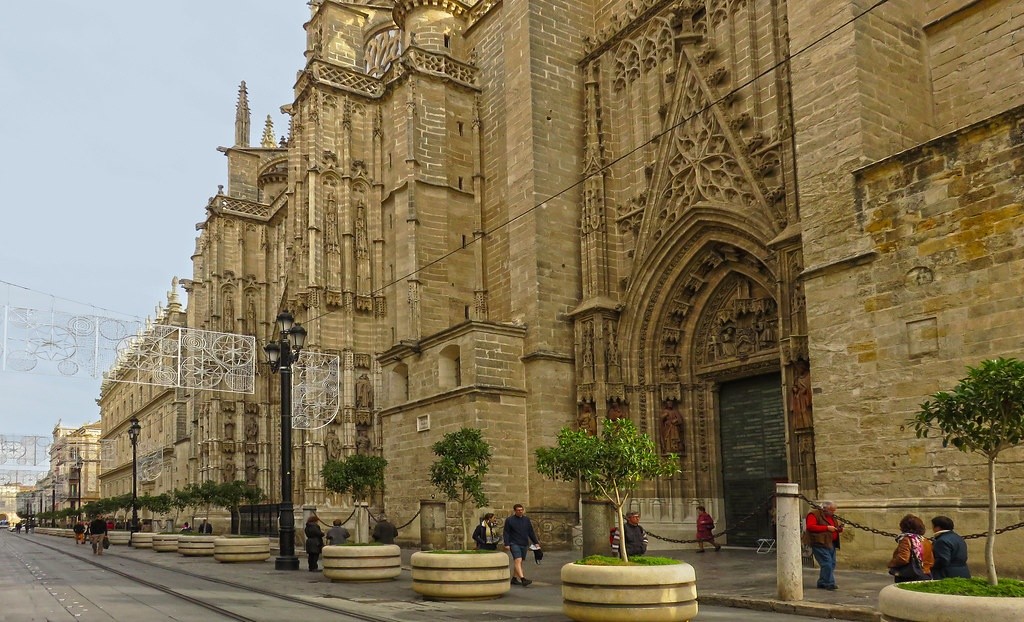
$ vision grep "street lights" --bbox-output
[262,306,308,572]
[76,458,84,522]
[127,414,141,547]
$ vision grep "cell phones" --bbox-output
[839,523,845,529]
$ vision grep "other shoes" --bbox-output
[715,545,721,552]
[510,577,522,585]
[817,582,839,591]
[696,548,705,553]
[522,579,532,586]
[310,567,323,572]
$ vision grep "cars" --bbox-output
[7,523,31,533]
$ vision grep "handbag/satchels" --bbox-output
[894,536,925,584]
[103,537,110,549]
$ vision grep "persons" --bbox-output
[304,516,325,571]
[612,510,648,559]
[14,521,30,535]
[578,404,597,434]
[804,502,844,591]
[889,515,934,584]
[471,513,500,552]
[608,402,626,421]
[792,361,813,430]
[73,518,89,546]
[694,504,723,553]
[661,400,683,453]
[504,503,539,587]
[929,516,973,580]
[89,516,109,557]
[180,522,189,532]
[371,515,399,544]
[325,519,351,545]
